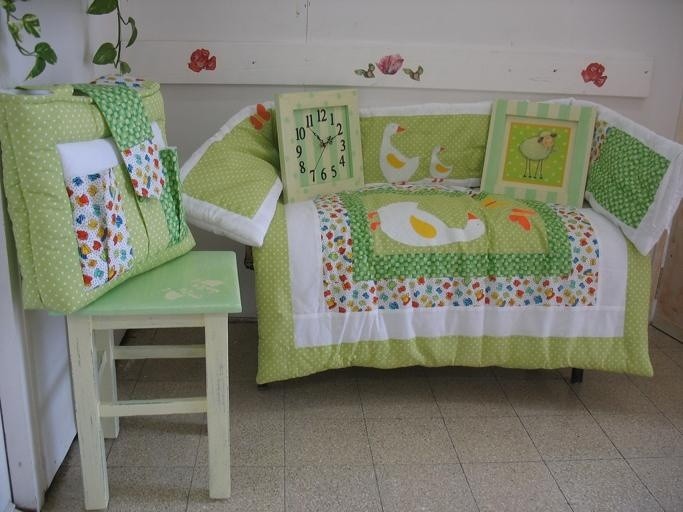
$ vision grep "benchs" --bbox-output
[177,94,679,387]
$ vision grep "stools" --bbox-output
[47,248,241,511]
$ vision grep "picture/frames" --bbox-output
[479,96,596,209]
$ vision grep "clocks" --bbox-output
[275,87,364,203]
